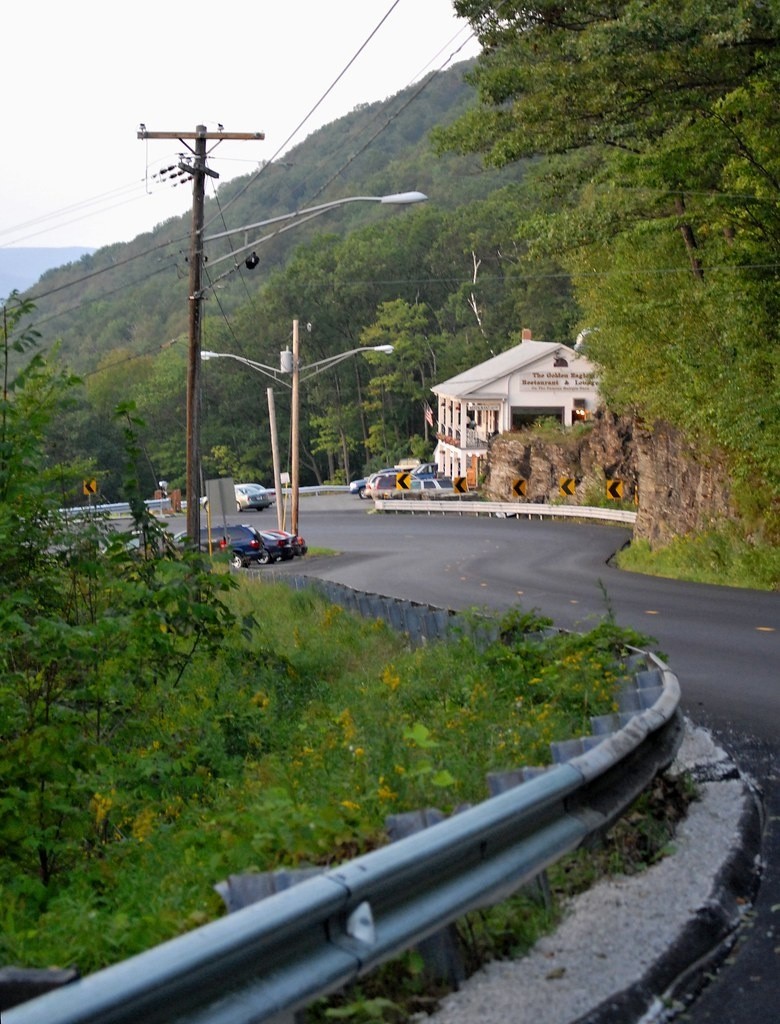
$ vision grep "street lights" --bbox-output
[184,190,430,632]
[199,320,396,536]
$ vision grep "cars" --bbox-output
[198,485,270,511]
[349,462,441,500]
[241,482,278,506]
[170,523,308,568]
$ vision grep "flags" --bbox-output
[425,401,434,427]
